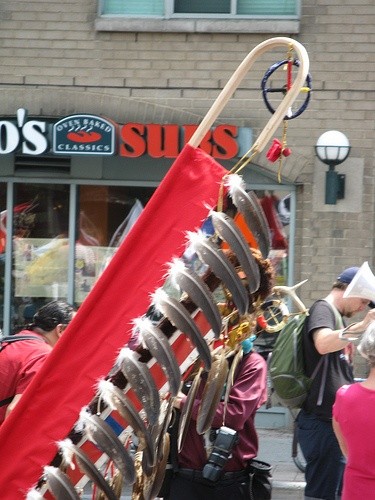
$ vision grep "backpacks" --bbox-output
[268,299,344,408]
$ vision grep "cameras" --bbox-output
[368,301,375,309]
[202,426,237,483]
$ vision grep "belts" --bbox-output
[178,468,250,485]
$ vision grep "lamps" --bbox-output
[314,129,353,205]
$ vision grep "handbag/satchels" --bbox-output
[236,460,272,500]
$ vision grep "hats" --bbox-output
[337,267,375,309]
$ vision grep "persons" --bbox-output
[270,266,371,500]
[331,320,374,499]
[0,299,78,425]
[130,323,272,499]
